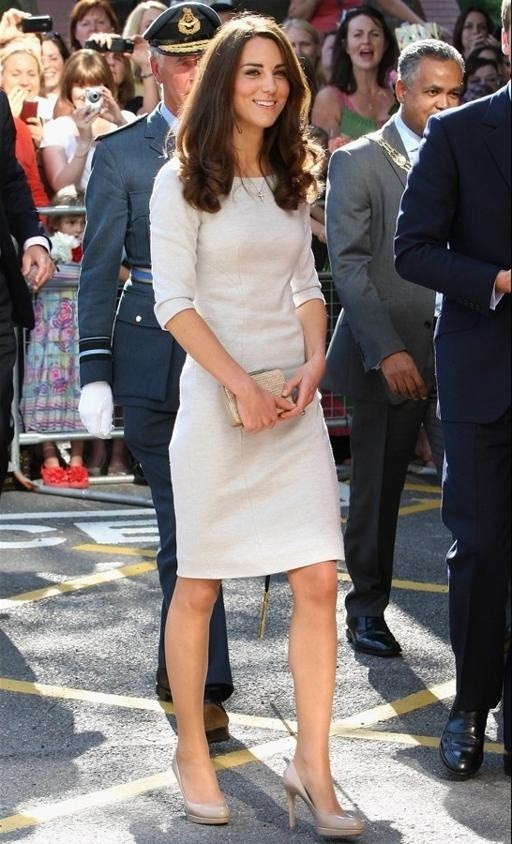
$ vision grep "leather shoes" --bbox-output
[439,706,489,776]
[346,617,401,655]
[151,667,230,704]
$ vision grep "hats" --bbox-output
[142,2,222,56]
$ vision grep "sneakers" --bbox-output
[40,463,70,488]
[66,465,89,489]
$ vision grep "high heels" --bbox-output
[85,449,106,476]
[282,761,365,836]
[173,749,230,824]
[108,449,132,475]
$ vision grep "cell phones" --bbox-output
[20,99,38,126]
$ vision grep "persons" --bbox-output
[77,1,236,746]
[388,0,512,778]
[322,35,472,658]
[144,9,365,844]
[2,0,511,488]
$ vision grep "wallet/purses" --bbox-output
[220,368,296,427]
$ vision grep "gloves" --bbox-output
[78,381,115,440]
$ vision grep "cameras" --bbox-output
[84,34,135,54]
[21,15,54,32]
[85,88,104,113]
